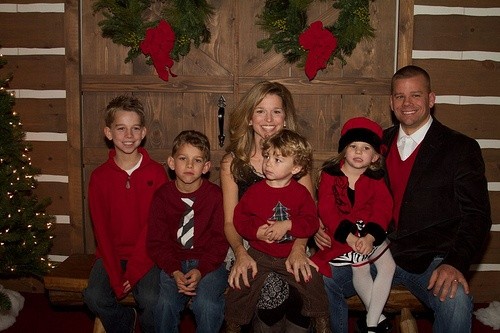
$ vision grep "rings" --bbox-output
[304,262,309,266]
[314,65,491,333]
[452,279,459,282]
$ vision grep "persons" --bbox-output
[145,130,230,333]
[221,129,331,333]
[83,95,168,333]
[309,117,396,333]
[219,81,319,333]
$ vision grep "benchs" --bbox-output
[45,252,425,333]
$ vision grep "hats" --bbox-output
[338,116,387,155]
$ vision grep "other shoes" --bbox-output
[357,316,378,333]
[378,313,392,333]
